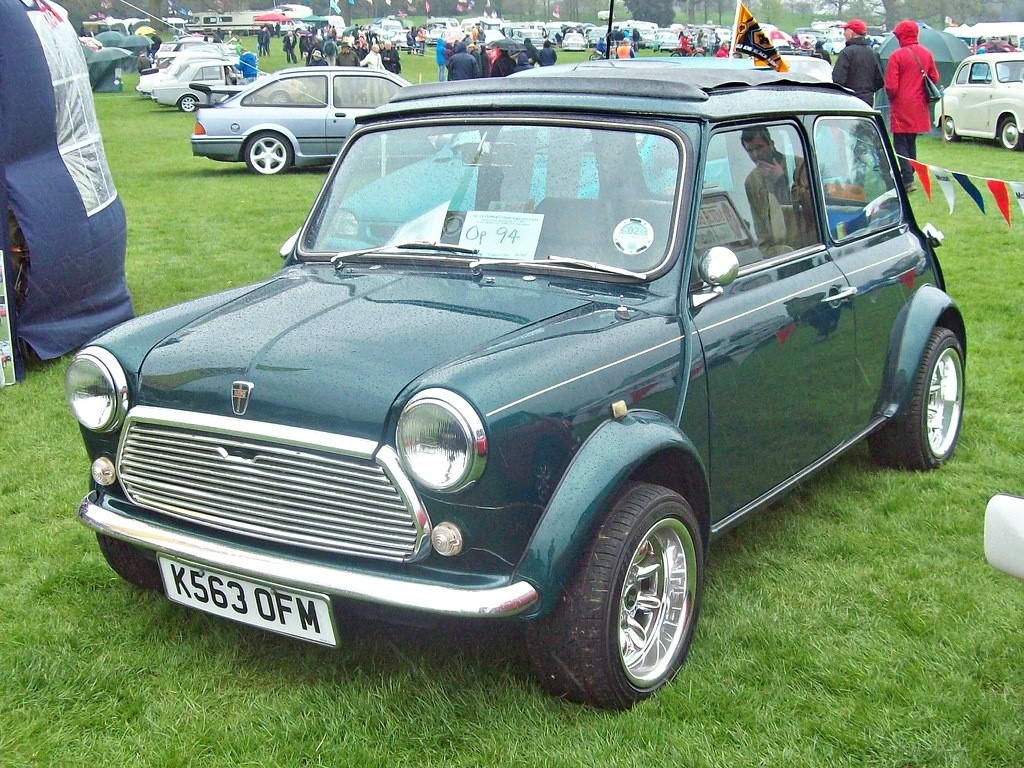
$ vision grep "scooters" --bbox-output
[670,40,706,58]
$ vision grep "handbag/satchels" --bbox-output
[904,46,942,103]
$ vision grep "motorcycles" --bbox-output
[589,47,617,61]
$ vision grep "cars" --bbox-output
[62,60,970,712]
[934,52,1024,151]
[190,64,414,175]
[134,15,1024,114]
[282,48,832,267]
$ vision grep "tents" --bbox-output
[875,29,974,135]
[0,0,133,383]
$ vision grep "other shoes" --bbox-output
[903,182,917,194]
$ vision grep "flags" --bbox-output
[734,3,790,72]
[946,17,959,26]
[553,4,559,18]
[330,0,498,20]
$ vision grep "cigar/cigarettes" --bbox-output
[758,163,762,168]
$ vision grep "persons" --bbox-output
[741,126,820,259]
[678,28,742,58]
[885,20,938,191]
[282,24,401,104]
[597,24,640,59]
[406,25,437,56]
[792,32,828,54]
[832,20,884,107]
[974,35,1024,81]
[436,24,584,82]
[204,23,281,85]
[137,35,179,76]
[79,26,95,37]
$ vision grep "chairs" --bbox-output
[531,195,612,261]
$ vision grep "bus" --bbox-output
[185,9,282,36]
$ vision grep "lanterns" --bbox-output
[255,14,329,32]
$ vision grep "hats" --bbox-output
[622,38,628,44]
[838,19,867,36]
[340,42,351,49]
[312,50,322,58]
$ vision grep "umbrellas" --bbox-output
[85,32,154,64]
[135,25,155,35]
